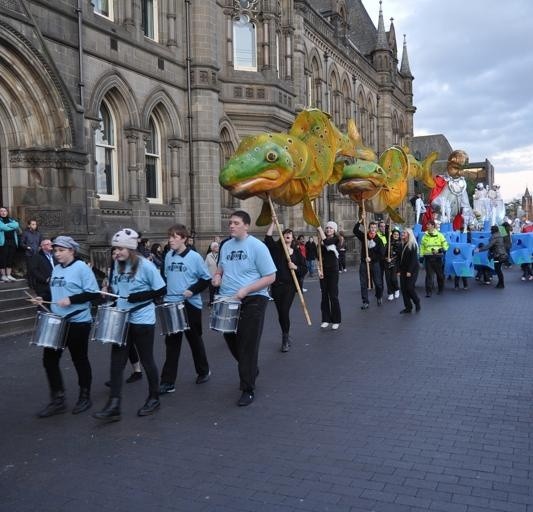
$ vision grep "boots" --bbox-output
[137,399,160,415]
[72,389,91,414]
[91,398,121,421]
[38,396,67,417]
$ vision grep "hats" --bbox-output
[326,221,337,233]
[111,228,139,250]
[51,236,79,255]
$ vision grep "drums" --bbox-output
[92,304,130,346]
[210,301,241,334]
[30,311,70,351]
[156,300,190,335]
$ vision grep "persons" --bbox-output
[420,149,481,232]
[0,208,42,282]
[315,213,531,331]
[29,211,318,422]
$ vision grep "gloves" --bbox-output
[420,257,423,263]
[438,248,443,253]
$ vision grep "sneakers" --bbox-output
[388,294,394,300]
[361,303,368,309]
[126,372,142,383]
[160,383,176,393]
[395,290,399,299]
[196,370,211,384]
[237,390,254,406]
[415,303,420,312]
[426,292,431,298]
[377,297,382,305]
[281,336,289,351]
[400,308,412,314]
[331,323,340,329]
[320,322,329,329]
